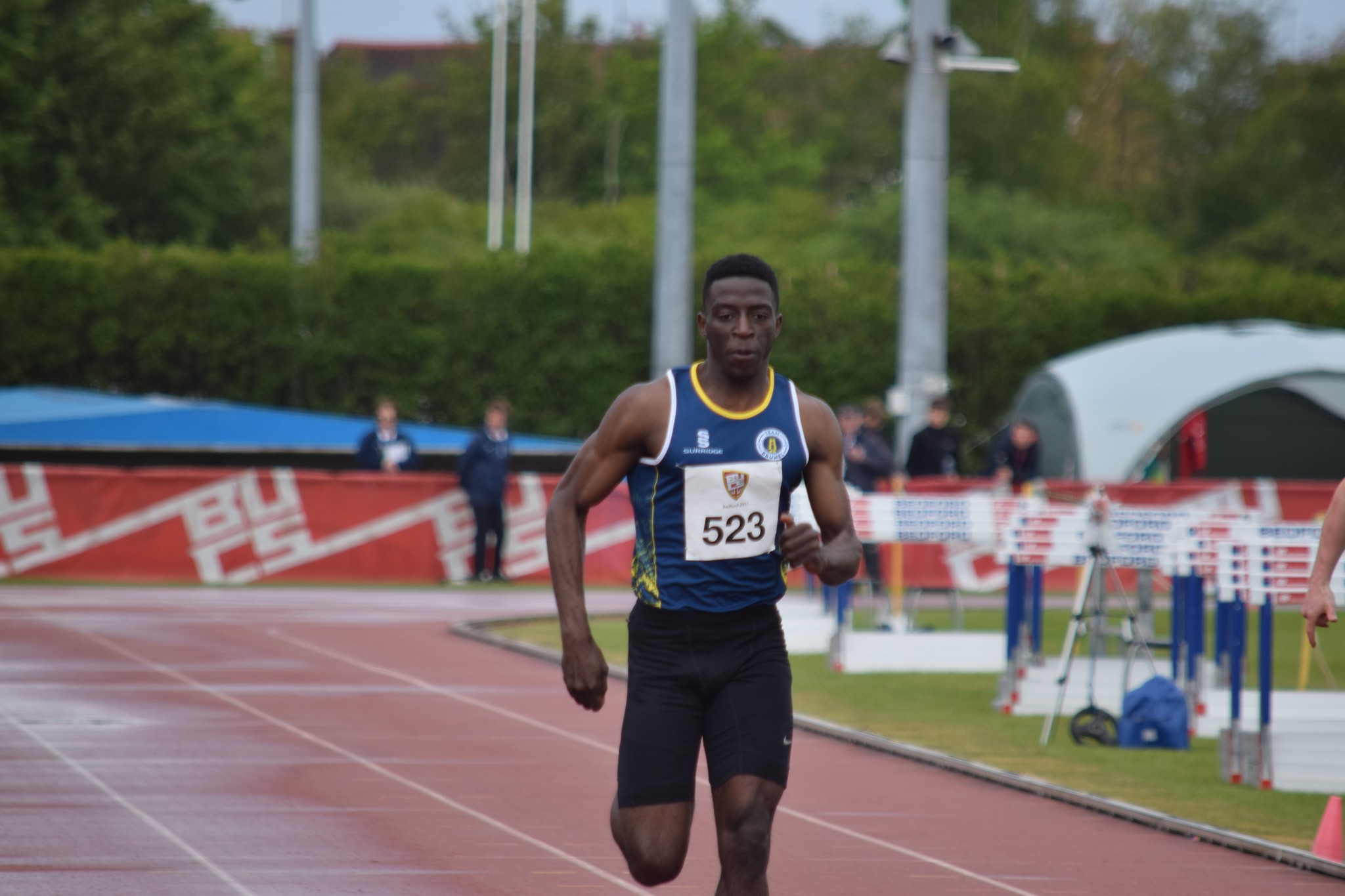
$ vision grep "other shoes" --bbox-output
[493,569,507,581]
[474,570,493,582]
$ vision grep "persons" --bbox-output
[545,253,860,896]
[1300,477,1345,647]
[836,391,1042,487]
[458,399,513,583]
[356,395,420,474]
